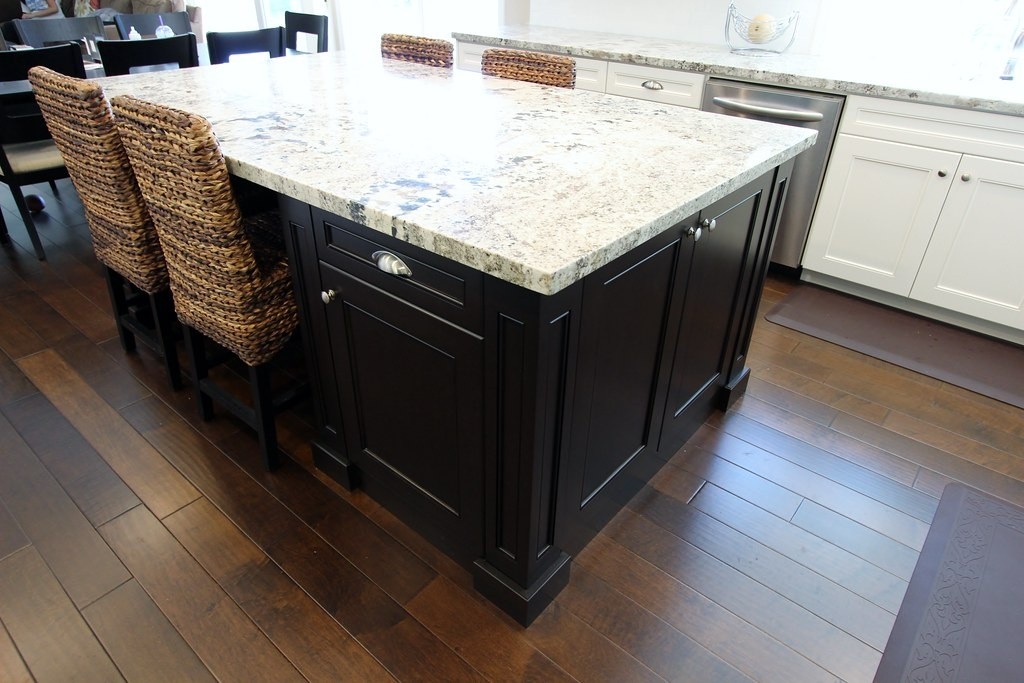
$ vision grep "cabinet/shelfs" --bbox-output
[455,41,705,109]
[800,95,1024,346]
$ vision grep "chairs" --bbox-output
[0,11,576,473]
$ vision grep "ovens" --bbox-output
[702,77,843,269]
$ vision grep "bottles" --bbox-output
[129,26,142,41]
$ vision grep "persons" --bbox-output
[22,0,66,19]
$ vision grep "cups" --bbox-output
[155,24,175,39]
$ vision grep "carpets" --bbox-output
[765,283,1024,410]
[873,482,1024,683]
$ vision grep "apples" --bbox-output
[747,14,776,43]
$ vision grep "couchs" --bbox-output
[0,0,204,50]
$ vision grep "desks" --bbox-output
[0,42,312,263]
[81,51,816,630]
